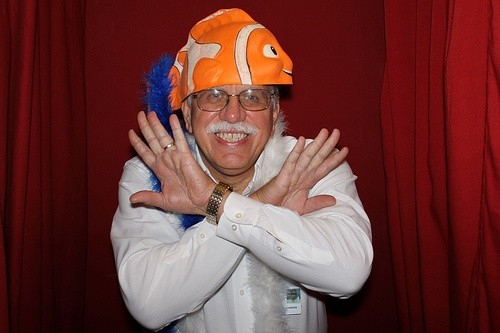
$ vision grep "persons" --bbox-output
[110,7,374,333]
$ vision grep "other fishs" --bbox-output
[168,8,294,111]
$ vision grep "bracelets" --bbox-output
[206,181,232,226]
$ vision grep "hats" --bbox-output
[142,8,293,108]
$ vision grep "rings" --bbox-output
[163,143,173,151]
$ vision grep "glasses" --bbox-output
[190,88,274,111]
